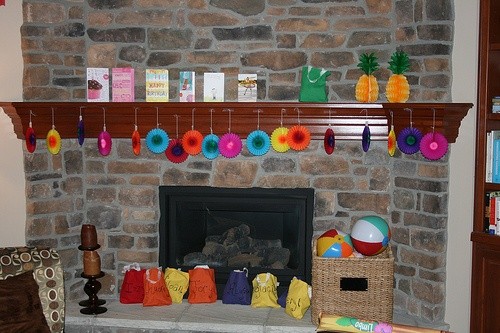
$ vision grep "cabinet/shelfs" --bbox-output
[470,0,500,333]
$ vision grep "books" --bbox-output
[481,95,500,236]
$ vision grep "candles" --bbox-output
[85,251,101,275]
[81,225,98,248]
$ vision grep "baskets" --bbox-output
[311,239,394,324]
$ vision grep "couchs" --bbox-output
[0,247,65,333]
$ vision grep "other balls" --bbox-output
[317,228,353,258]
[351,216,391,256]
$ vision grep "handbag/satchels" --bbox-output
[278,288,287,308]
[251,273,281,308]
[285,276,312,320]
[120,263,146,304]
[143,266,172,307]
[222,268,251,305]
[188,265,217,303]
[164,267,189,304]
[298,65,331,102]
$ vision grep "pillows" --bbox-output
[0,270,51,333]
